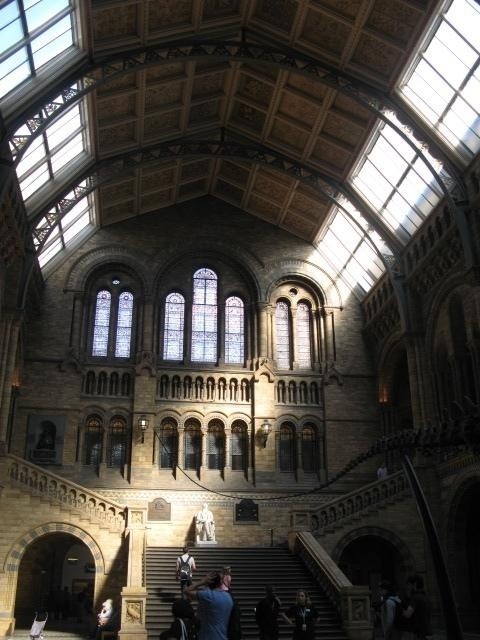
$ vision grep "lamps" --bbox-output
[138,414,150,444]
[260,419,273,448]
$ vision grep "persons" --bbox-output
[371,582,428,640]
[159,567,236,640]
[49,585,91,624]
[377,462,387,480]
[176,547,196,602]
[196,503,214,540]
[255,584,320,640]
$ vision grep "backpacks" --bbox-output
[179,556,191,581]
[389,598,410,631]
